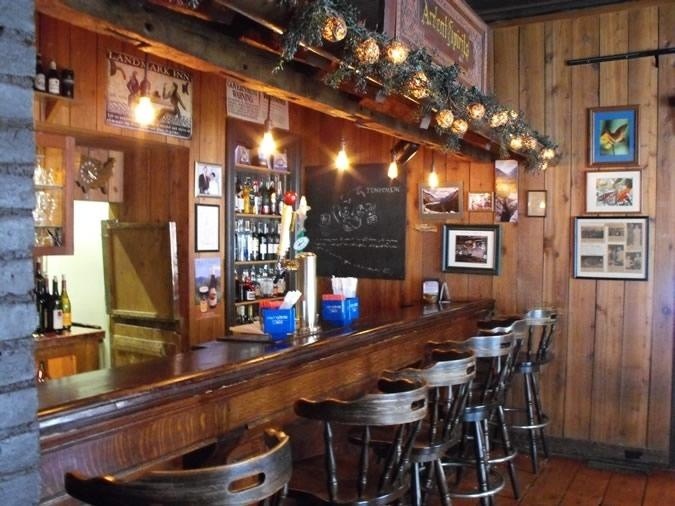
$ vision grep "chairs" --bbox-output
[380,347,476,506]
[208,427,290,505]
[474,317,528,498]
[488,307,558,474]
[291,374,428,505]
[425,330,516,506]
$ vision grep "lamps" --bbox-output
[535,159,548,171]
[357,35,380,64]
[387,136,399,179]
[451,118,468,134]
[436,109,454,128]
[382,39,408,65]
[407,71,432,98]
[510,136,523,150]
[522,135,538,148]
[427,148,440,189]
[466,102,485,121]
[336,120,350,170]
[259,94,277,155]
[490,108,519,127]
[133,51,157,126]
[321,16,347,41]
[537,148,555,160]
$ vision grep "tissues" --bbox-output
[262,291,303,339]
[323,273,360,321]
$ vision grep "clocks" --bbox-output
[80,161,98,183]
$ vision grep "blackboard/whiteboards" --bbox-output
[303,162,406,281]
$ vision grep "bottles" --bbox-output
[47,60,60,95]
[60,279,71,329]
[37,280,48,332]
[35,262,42,292]
[48,279,63,333]
[199,287,209,313]
[34,53,46,91]
[208,274,217,309]
[237,305,253,325]
[62,64,74,98]
[234,263,287,302]
[43,278,51,301]
[235,176,284,216]
[234,218,280,261]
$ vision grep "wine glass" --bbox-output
[33,191,56,226]
[33,155,47,185]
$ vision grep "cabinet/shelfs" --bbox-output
[38,297,494,505]
[33,130,74,255]
[33,324,107,383]
[225,115,301,335]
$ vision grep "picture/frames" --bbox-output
[572,215,650,280]
[584,167,642,213]
[417,179,464,219]
[466,190,494,212]
[194,159,223,199]
[526,189,547,217]
[438,223,502,275]
[494,159,519,223]
[194,202,220,252]
[586,103,640,167]
